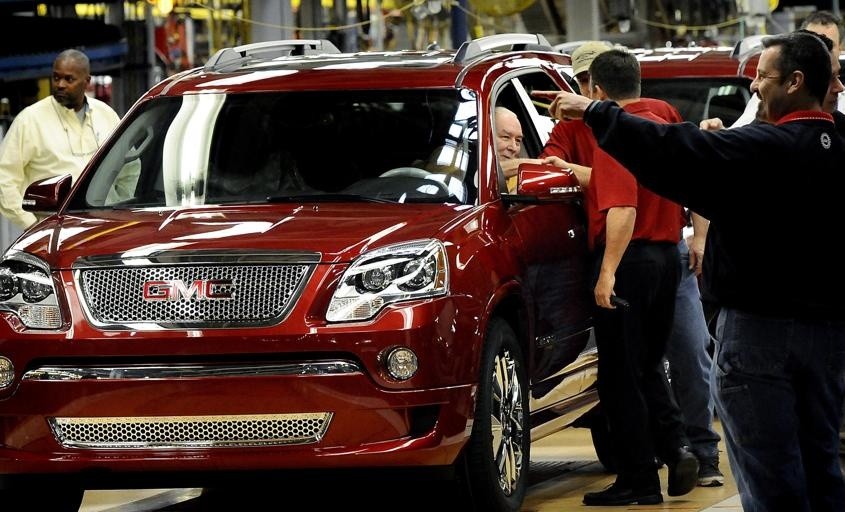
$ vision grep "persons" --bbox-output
[326,16,345,53]
[0,48,142,232]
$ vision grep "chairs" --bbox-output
[207,105,448,199]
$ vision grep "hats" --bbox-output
[569,42,608,81]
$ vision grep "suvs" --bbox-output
[0,28,664,511]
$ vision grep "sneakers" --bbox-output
[698,453,724,487]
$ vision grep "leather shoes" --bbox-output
[583,483,663,505]
[667,447,699,496]
